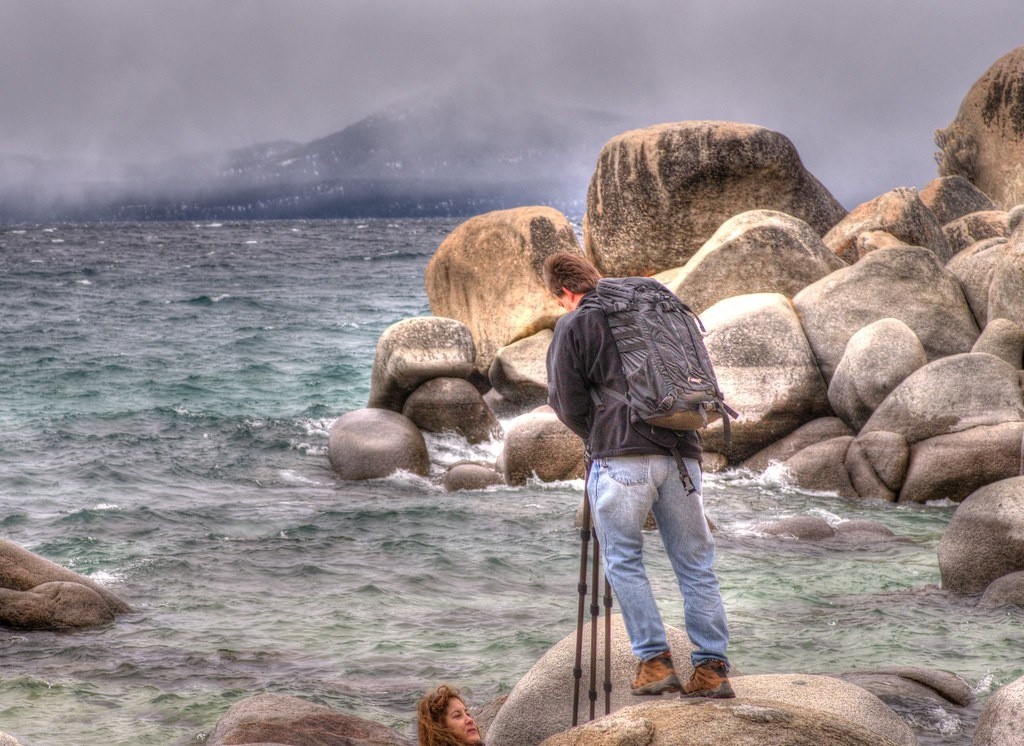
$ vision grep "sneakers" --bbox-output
[629,651,682,696]
[680,661,735,698]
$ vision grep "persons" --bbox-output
[418,685,485,746]
[543,252,735,698]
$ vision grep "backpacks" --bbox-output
[574,277,738,455]
